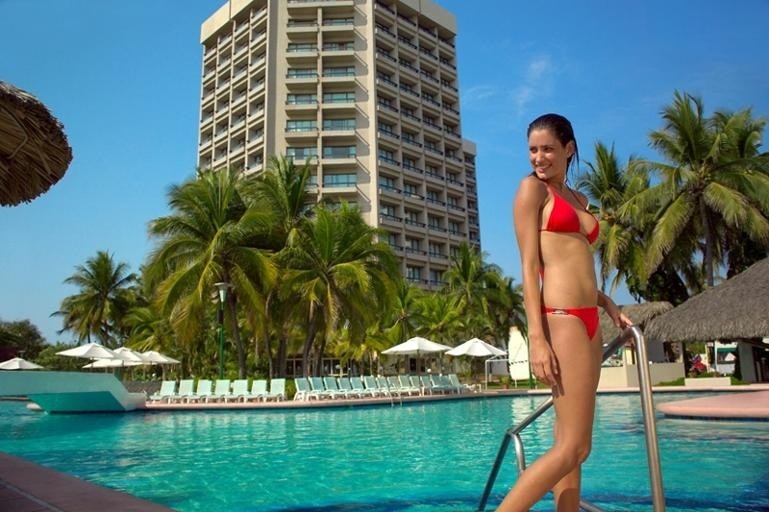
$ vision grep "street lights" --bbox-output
[212,280,234,382]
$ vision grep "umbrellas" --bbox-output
[443,336,509,384]
[0,356,45,371]
[54,341,182,382]
[380,335,454,375]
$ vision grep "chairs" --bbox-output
[149,379,288,403]
[292,373,468,401]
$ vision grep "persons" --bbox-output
[688,355,707,375]
[487,113,636,511]
